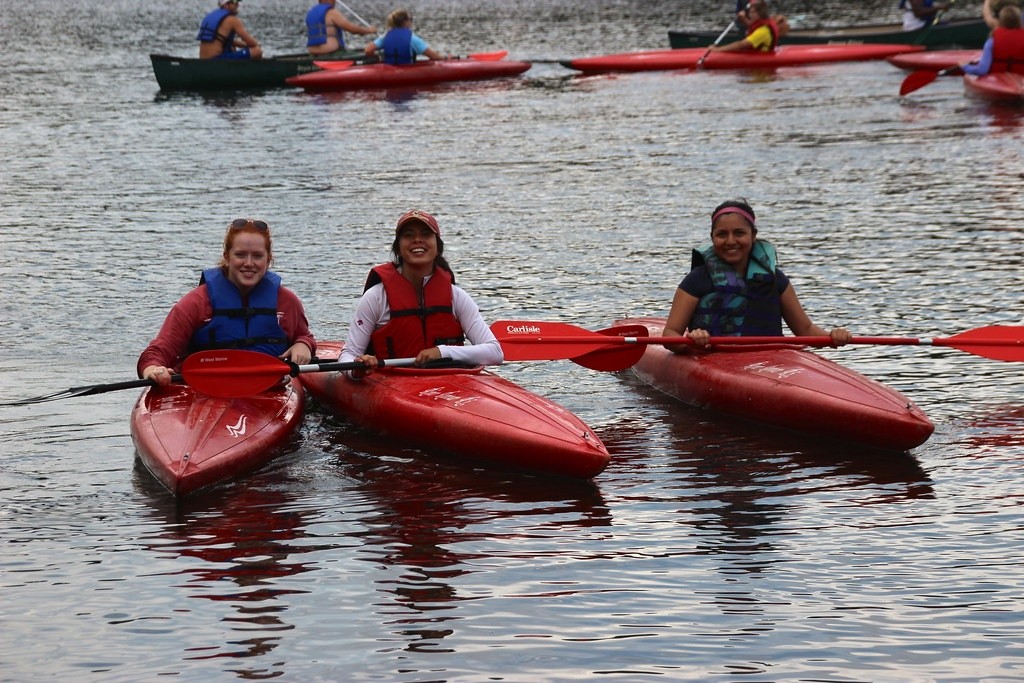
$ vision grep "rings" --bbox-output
[160,372,163,374]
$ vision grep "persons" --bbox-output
[708,0,779,53]
[982,0,1024,37]
[899,0,946,32]
[959,6,1024,75]
[306,0,377,55]
[364,7,454,65]
[195,0,263,61]
[337,210,504,381]
[660,195,853,352]
[135,217,318,387]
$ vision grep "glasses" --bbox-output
[229,218,269,232]
[407,17,413,22]
[229,0,238,4]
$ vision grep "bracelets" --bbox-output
[443,53,448,59]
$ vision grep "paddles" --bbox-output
[180,321,650,412]
[914,0,957,45]
[899,60,975,97]
[336,0,383,37]
[313,49,511,70]
[37,357,336,404]
[490,318,1024,363]
[688,0,758,71]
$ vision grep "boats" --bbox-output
[130,358,307,503]
[612,317,935,451]
[556,45,928,70]
[885,49,984,77]
[149,47,384,92]
[296,339,613,481]
[961,70,1024,104]
[284,59,532,88]
[667,15,993,50]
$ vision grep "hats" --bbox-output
[218,0,230,8]
[397,210,441,239]
